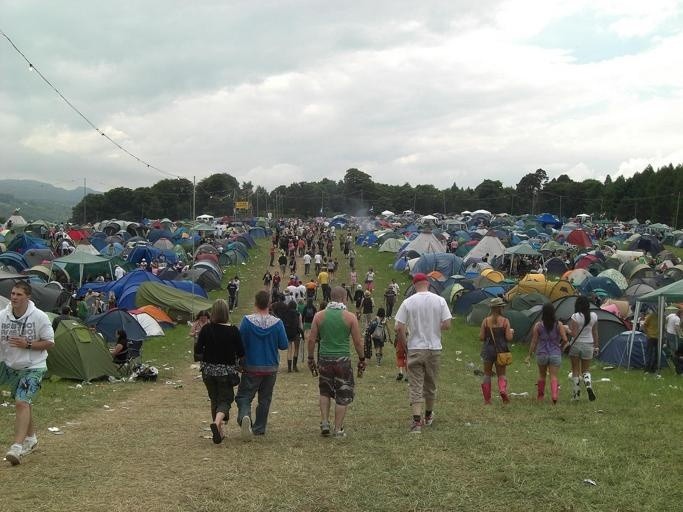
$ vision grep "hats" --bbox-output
[490,297,506,306]
[413,273,428,283]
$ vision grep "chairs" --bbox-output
[112,347,136,374]
[127,339,143,366]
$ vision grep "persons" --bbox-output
[1,282,54,468]
[226,214,409,383]
[563,294,601,404]
[479,296,514,404]
[235,289,289,442]
[192,299,247,444]
[524,302,568,403]
[305,285,367,437]
[394,272,451,438]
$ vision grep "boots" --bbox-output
[583,372,596,401]
[498,376,509,402]
[570,377,580,400]
[481,383,492,404]
[551,380,557,403]
[538,380,545,401]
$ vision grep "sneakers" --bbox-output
[21,438,39,457]
[5,446,21,465]
[241,416,253,441]
[408,422,421,434]
[333,429,346,437]
[320,421,330,433]
[210,423,221,445]
[425,412,433,426]
[226,373,239,386]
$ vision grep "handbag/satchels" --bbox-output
[496,353,513,365]
[563,341,570,353]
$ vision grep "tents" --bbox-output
[326,209,408,253]
[0,206,275,386]
[394,210,683,372]
